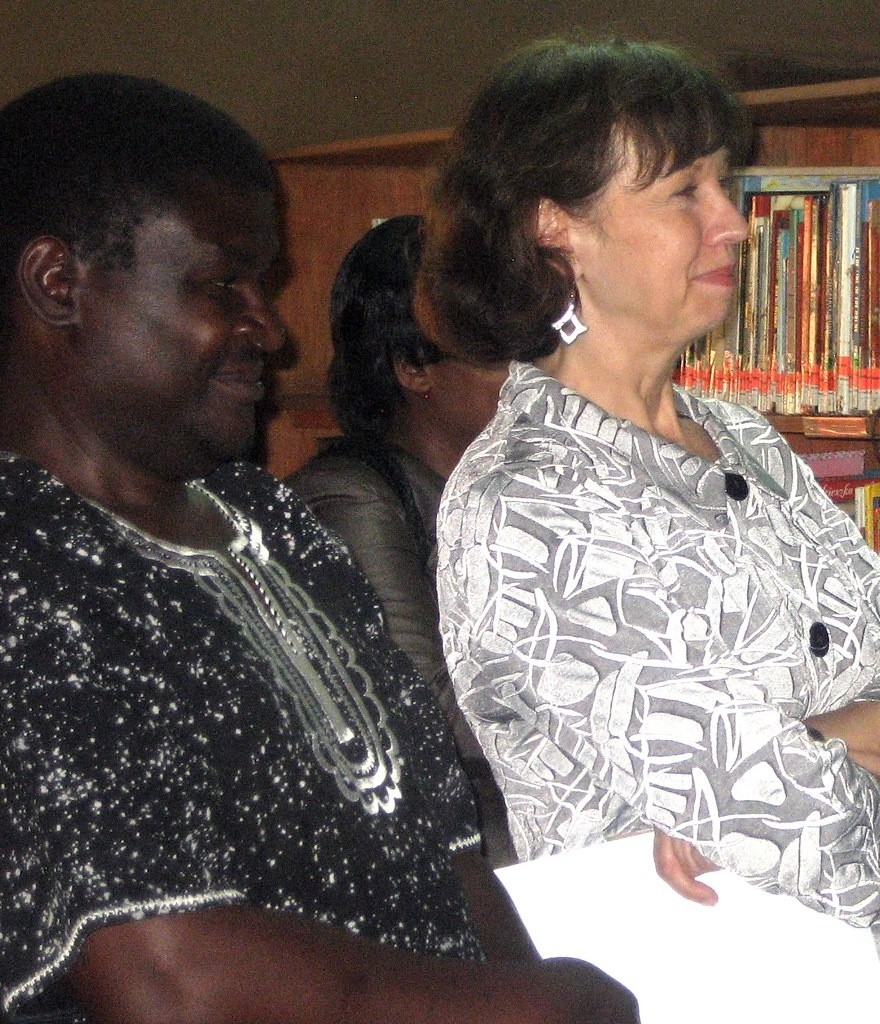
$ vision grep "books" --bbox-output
[673,166,880,554]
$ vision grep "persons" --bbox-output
[275,216,513,793]
[414,39,880,934]
[0,72,639,1024]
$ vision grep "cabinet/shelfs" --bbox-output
[254,79,878,555]
